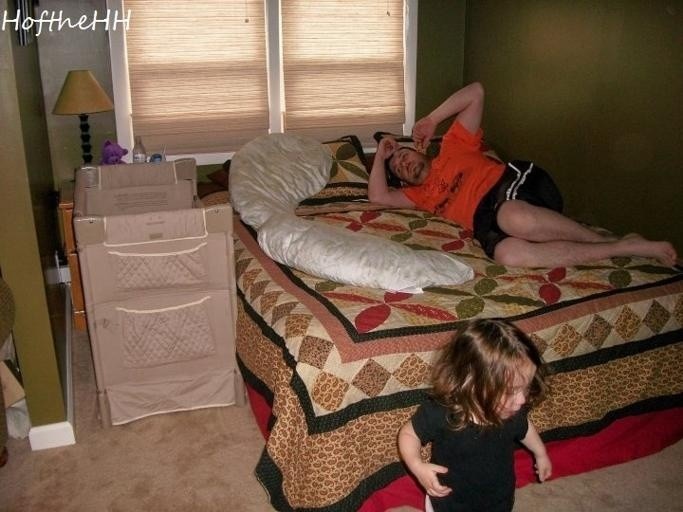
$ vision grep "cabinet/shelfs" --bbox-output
[74,157,241,428]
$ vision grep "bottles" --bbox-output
[132,136,147,162]
[150,154,162,163]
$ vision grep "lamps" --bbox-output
[49,69,114,183]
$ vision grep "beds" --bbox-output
[191,154,682,502]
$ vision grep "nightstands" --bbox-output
[56,179,90,332]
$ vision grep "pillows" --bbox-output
[294,130,442,206]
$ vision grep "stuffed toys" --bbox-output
[99,140,130,165]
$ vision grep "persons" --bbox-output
[397,316,554,512]
[369,82,677,268]
